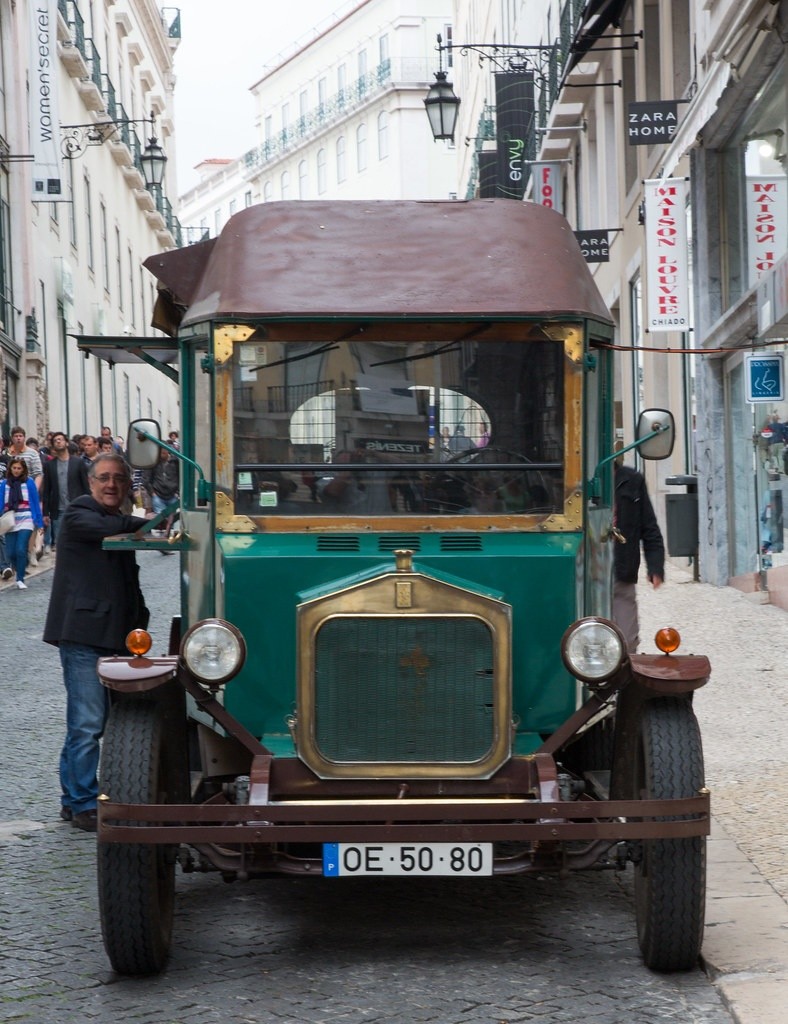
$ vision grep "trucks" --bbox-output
[66,202,711,974]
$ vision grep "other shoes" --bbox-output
[60,805,74,821]
[27,553,38,567]
[2,568,12,580]
[72,808,98,832]
[16,581,27,590]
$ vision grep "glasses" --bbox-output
[92,474,128,484]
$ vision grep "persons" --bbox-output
[449,424,479,466]
[40,452,174,834]
[756,412,785,552]
[0,457,44,591]
[475,422,490,448]
[613,460,666,658]
[0,425,181,581]
[233,433,565,516]
[441,426,452,450]
